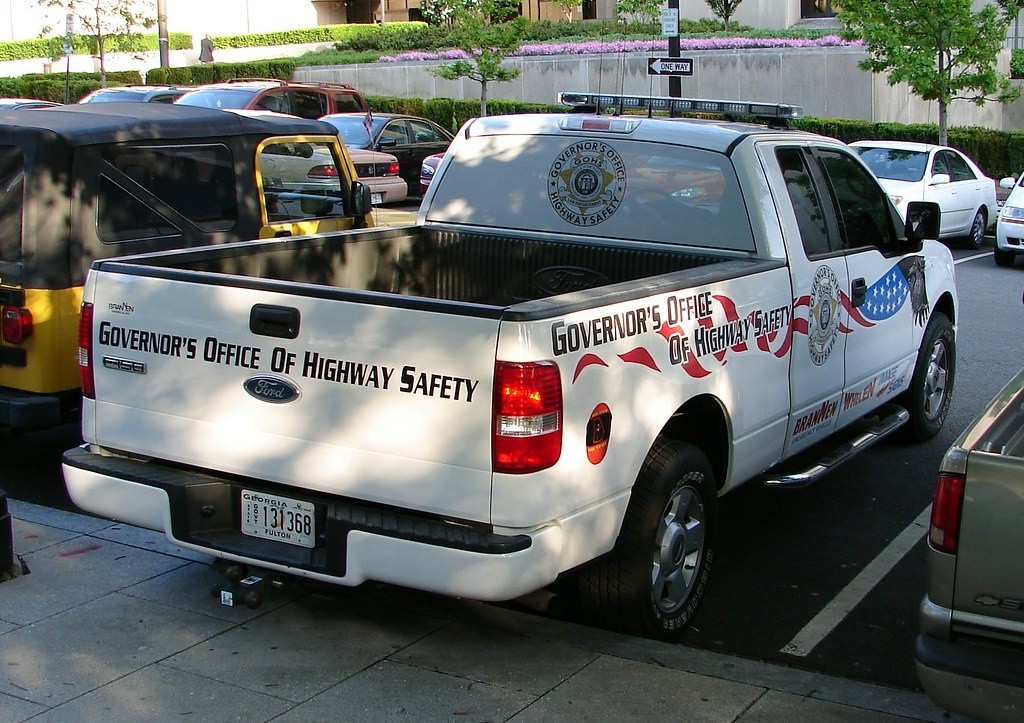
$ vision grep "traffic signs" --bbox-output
[647,57,695,77]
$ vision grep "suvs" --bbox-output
[79,84,197,105]
[0,102,419,485]
[172,77,368,121]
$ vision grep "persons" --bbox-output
[198,33,216,64]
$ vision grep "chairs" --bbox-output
[887,160,909,174]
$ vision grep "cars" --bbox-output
[846,140,998,249]
[317,112,455,198]
[223,109,408,217]
[995,171,1024,266]
[623,157,725,211]
[419,152,448,201]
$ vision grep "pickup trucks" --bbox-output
[916,363,1024,723]
[61,92,959,640]
